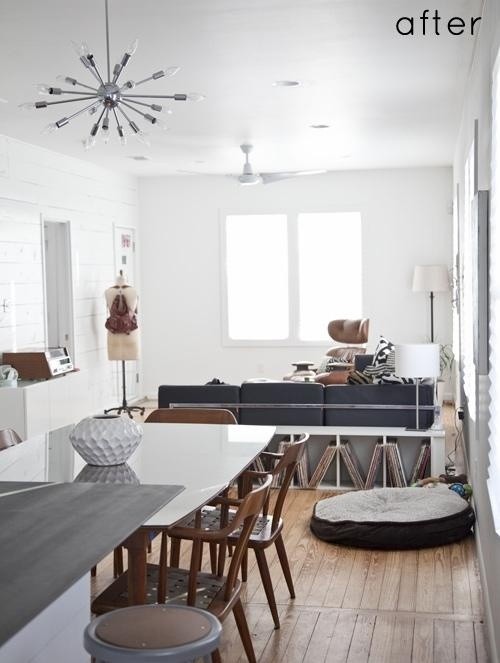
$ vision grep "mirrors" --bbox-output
[40,215,75,370]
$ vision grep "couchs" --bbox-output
[158,381,435,430]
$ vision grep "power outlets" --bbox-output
[459,407,465,419]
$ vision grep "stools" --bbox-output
[84,604,223,663]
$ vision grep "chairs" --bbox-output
[92,473,274,663]
[1,427,99,577]
[167,433,310,629]
[315,318,368,385]
[117,409,238,576]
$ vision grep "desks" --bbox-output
[0,421,277,606]
[0,482,184,663]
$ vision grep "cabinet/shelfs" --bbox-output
[1,370,83,483]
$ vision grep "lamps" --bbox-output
[413,266,449,342]
[394,343,439,431]
[20,1,205,154]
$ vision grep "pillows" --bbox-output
[372,336,395,367]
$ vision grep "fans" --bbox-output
[179,143,327,196]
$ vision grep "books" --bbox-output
[252,439,431,488]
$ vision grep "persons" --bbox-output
[104,276,139,360]
